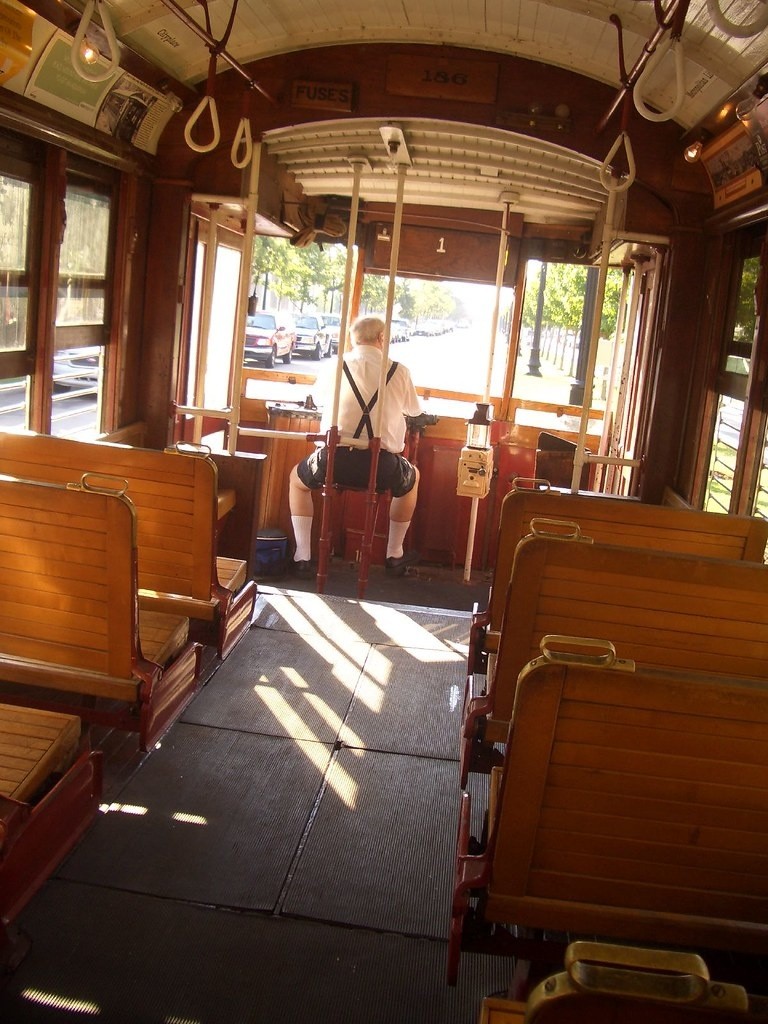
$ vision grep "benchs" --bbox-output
[0,429,768,1024]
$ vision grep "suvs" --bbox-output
[244,310,298,370]
[294,314,334,362]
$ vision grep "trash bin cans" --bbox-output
[256,527,288,577]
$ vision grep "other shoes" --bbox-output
[288,557,314,579]
[384,549,420,575]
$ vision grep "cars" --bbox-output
[52,345,105,399]
[322,313,342,354]
[390,319,455,344]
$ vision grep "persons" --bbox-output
[287,317,420,579]
